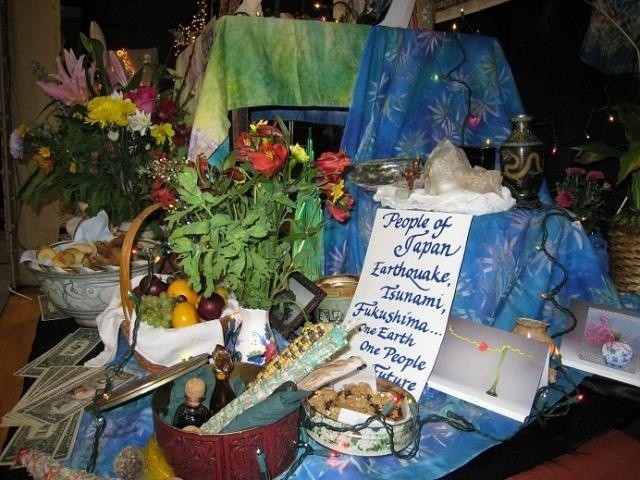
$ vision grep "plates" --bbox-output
[347,157,426,193]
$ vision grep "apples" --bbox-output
[197,292,225,321]
[132,287,141,296]
[140,275,163,296]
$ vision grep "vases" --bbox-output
[509,316,556,359]
[600,341,635,371]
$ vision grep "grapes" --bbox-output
[178,295,186,302]
[133,291,177,328]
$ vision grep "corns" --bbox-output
[248,321,332,386]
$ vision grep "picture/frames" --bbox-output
[266,267,329,341]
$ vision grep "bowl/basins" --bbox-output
[300,374,421,457]
[27,239,167,331]
[151,360,300,480]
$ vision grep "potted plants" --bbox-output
[568,97,639,296]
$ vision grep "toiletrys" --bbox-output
[209,343,236,416]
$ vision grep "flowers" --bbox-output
[584,310,622,349]
[550,160,614,226]
[148,114,357,337]
[3,18,201,235]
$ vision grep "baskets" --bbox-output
[119,203,234,376]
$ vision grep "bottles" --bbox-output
[208,367,236,415]
